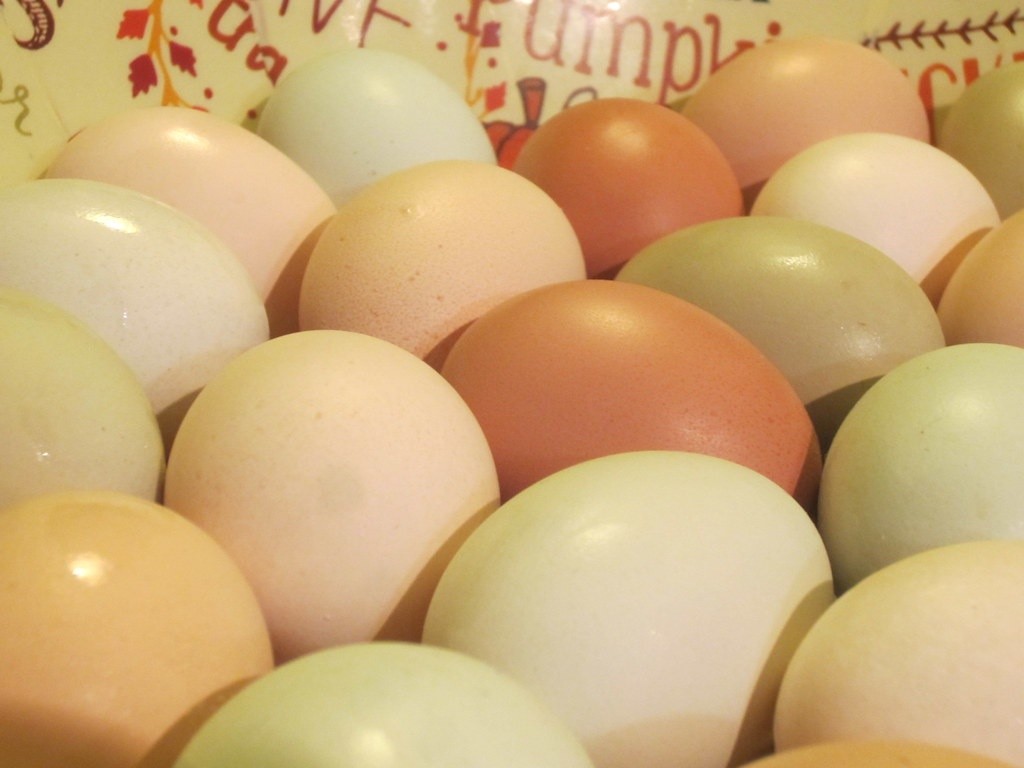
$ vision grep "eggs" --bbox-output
[0,33,1024,768]
